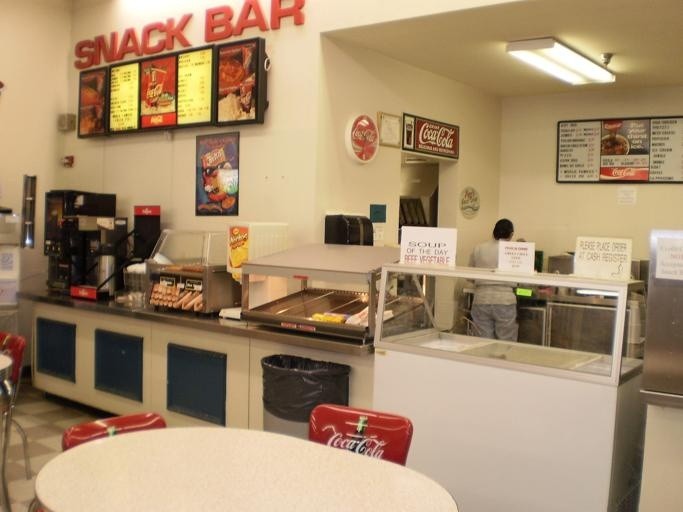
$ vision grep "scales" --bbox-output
[514,287,535,297]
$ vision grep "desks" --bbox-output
[35,426,461,512]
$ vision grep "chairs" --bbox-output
[307,402,410,467]
[62,412,166,450]
[0,330,31,511]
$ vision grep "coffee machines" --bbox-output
[42,189,129,300]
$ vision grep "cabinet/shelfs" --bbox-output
[371,264,648,512]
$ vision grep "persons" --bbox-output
[464,217,519,342]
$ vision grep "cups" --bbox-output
[405,122,413,144]
[145,66,167,108]
[238,81,253,112]
[601,133,630,156]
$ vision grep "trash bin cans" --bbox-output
[261,355,350,439]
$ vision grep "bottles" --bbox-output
[348,417,368,455]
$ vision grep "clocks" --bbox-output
[344,114,379,164]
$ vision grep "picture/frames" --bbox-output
[377,111,402,150]
